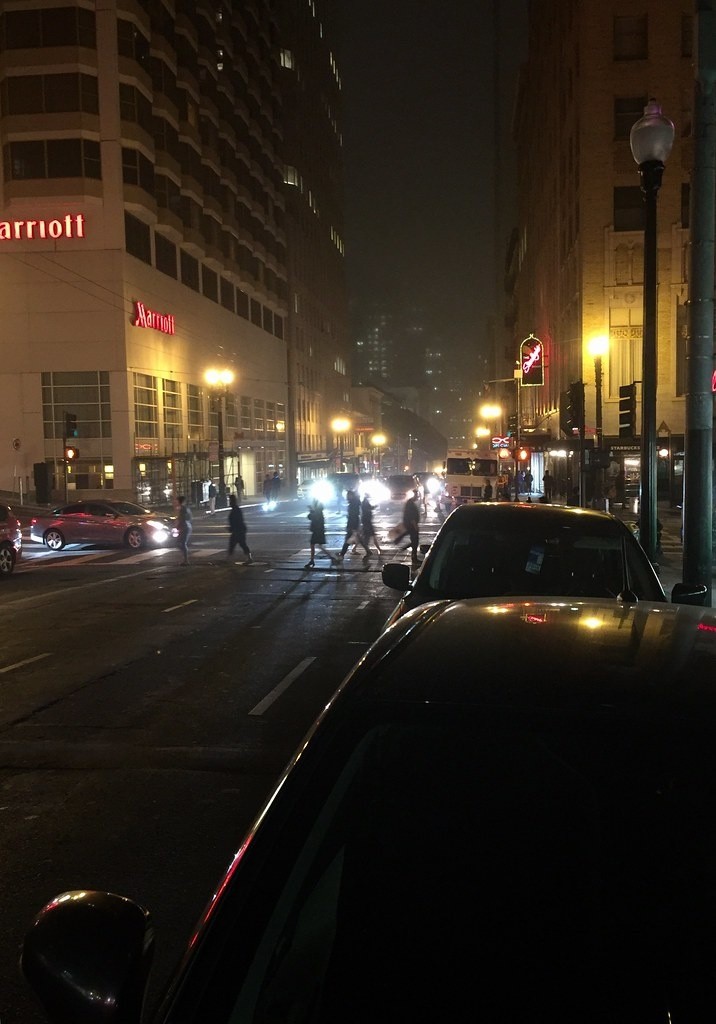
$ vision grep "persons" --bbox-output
[209,479,216,515]
[263,472,280,506]
[305,498,337,567]
[567,487,579,506]
[509,470,532,494]
[336,491,386,562]
[402,489,422,563]
[222,495,253,564]
[484,480,492,498]
[543,470,553,499]
[616,470,629,509]
[171,496,193,567]
[235,476,244,497]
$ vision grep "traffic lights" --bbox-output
[619,384,636,438]
[66,447,79,461]
[565,382,584,433]
[508,415,518,437]
[520,436,529,461]
[64,414,78,438]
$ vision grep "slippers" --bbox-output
[350,552,361,554]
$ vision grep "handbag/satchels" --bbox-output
[346,532,359,544]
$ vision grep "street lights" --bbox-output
[626,98,675,553]
[205,368,234,509]
[331,417,349,471]
[480,405,500,448]
[587,330,608,446]
[374,435,386,475]
[407,434,417,466]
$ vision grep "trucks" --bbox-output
[442,448,498,509]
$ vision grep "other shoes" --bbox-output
[305,561,315,566]
[243,559,254,565]
[377,550,386,555]
[412,558,423,563]
[364,553,373,557]
[181,561,190,566]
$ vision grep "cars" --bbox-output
[28,498,181,551]
[296,470,442,503]
[20,596,716,1024]
[0,501,24,575]
[382,501,710,607]
